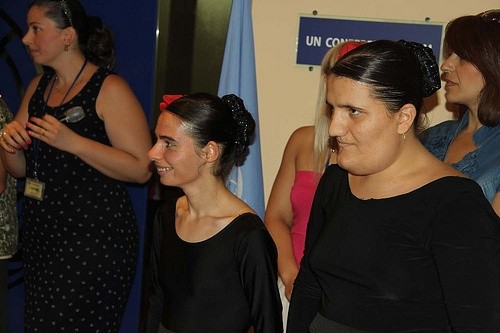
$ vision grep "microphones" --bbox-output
[56,107,87,123]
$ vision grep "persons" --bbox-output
[263,42,368,333]
[143,93,284,333]
[286,40,500,333]
[418,8,500,217]
[0,0,153,333]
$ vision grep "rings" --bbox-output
[0,132,7,136]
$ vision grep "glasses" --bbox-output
[477,10,500,23]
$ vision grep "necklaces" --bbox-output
[330,146,336,155]
